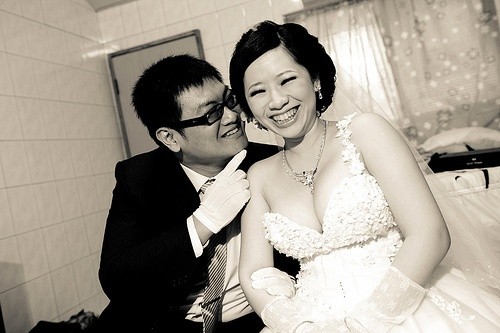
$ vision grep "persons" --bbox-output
[94,55,301,333]
[229,21,500,333]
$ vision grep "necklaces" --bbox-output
[282,121,327,191]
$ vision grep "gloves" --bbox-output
[251,266,296,297]
[193,149,251,234]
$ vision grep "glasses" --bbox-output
[174,89,239,131]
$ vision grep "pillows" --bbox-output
[422,127,500,154]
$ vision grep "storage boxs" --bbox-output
[440,148,500,170]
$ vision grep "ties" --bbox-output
[200,179,227,332]
